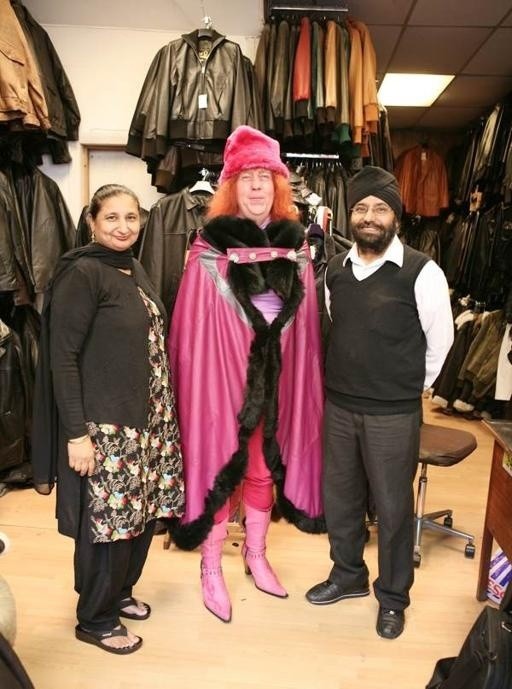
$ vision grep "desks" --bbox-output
[473,417,512,607]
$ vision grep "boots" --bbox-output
[199,514,233,623]
[241,498,289,599]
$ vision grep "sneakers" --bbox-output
[305,579,370,605]
[376,608,404,639]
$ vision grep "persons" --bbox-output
[305,166,454,639]
[35,184,185,655]
[166,125,326,623]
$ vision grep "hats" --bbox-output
[345,165,403,219]
[218,125,291,187]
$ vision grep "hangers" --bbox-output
[194,12,221,43]
[281,152,346,177]
[186,167,216,201]
[268,1,351,23]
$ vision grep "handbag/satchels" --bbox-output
[425,604,512,689]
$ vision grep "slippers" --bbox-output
[75,623,144,655]
[118,596,151,620]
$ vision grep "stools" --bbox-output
[362,419,483,571]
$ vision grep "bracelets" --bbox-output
[68,435,90,444]
